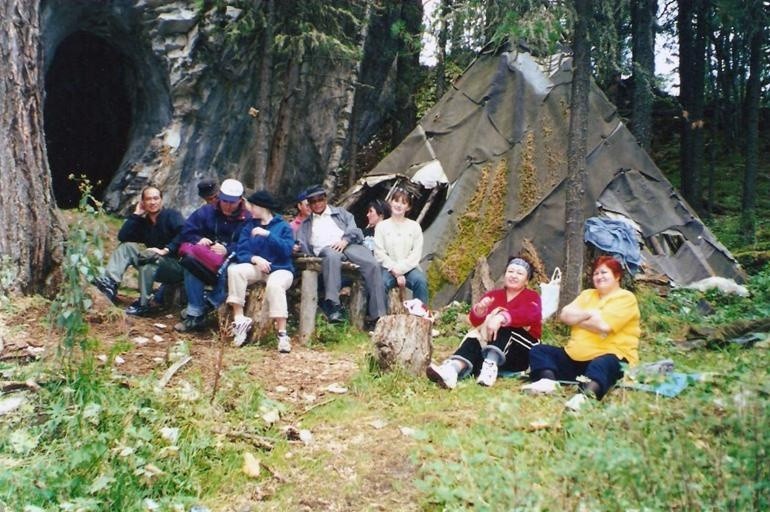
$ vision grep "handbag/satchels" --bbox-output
[179,241,229,285]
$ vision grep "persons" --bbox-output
[426,258,541,390]
[521,256,641,412]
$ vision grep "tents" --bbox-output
[331,34,752,313]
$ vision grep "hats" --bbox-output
[307,185,326,201]
[298,190,307,201]
[198,180,219,197]
[220,178,244,202]
[247,189,281,209]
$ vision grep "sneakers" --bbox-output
[564,394,587,411]
[126,307,149,317]
[91,277,115,301]
[175,300,213,331]
[477,360,498,386]
[231,316,254,348]
[428,363,458,391]
[521,378,559,395]
[330,311,344,323]
[277,335,292,354]
